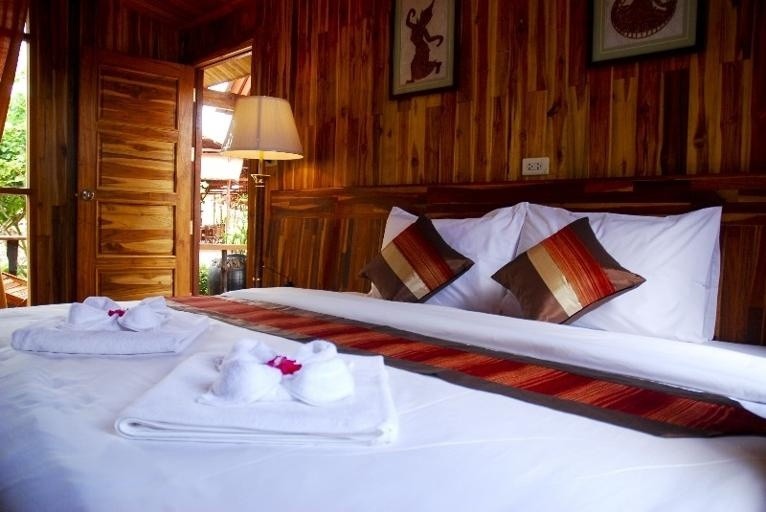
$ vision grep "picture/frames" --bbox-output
[586,0,711,69]
[389,0,466,100]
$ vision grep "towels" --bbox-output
[66,292,172,333]
[209,339,357,407]
[111,351,403,448]
[10,310,210,353]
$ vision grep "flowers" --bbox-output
[267,353,303,377]
[105,307,129,322]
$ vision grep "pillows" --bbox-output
[361,214,475,306]
[488,217,646,324]
[368,200,527,315]
[496,203,725,344]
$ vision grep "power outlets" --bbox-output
[523,157,551,175]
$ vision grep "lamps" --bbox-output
[220,92,308,288]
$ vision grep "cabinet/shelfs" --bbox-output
[199,147,240,293]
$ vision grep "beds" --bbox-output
[0,183,765,510]
